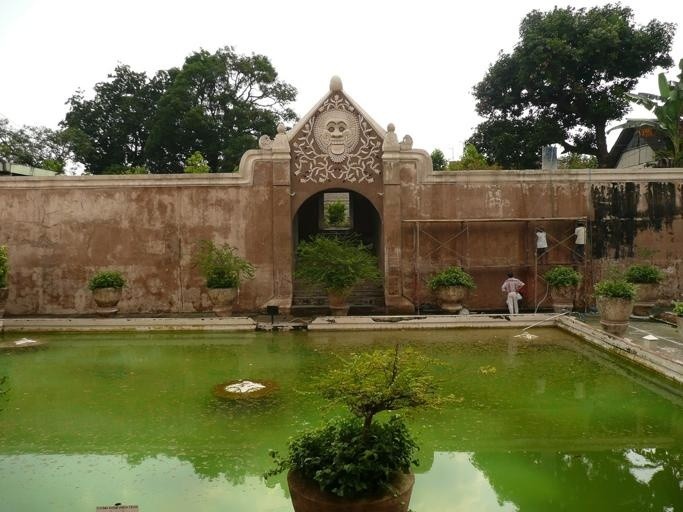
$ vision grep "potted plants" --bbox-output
[0,245,11,318]
[87,270,129,317]
[428,262,477,313]
[190,239,256,317]
[261,410,423,511]
[292,232,384,317]
[543,260,683,339]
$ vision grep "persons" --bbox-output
[570,222,587,263]
[535,227,549,265]
[500,271,527,314]
